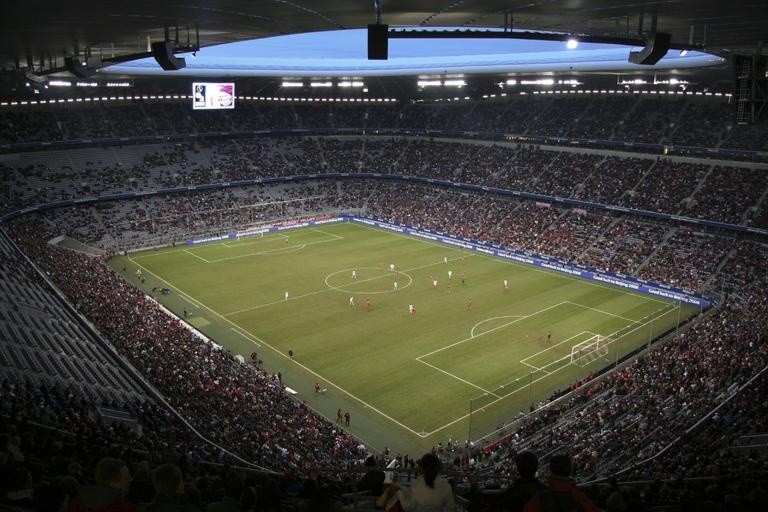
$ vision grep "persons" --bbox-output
[1,95,768,512]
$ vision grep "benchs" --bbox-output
[0,228,150,409]
